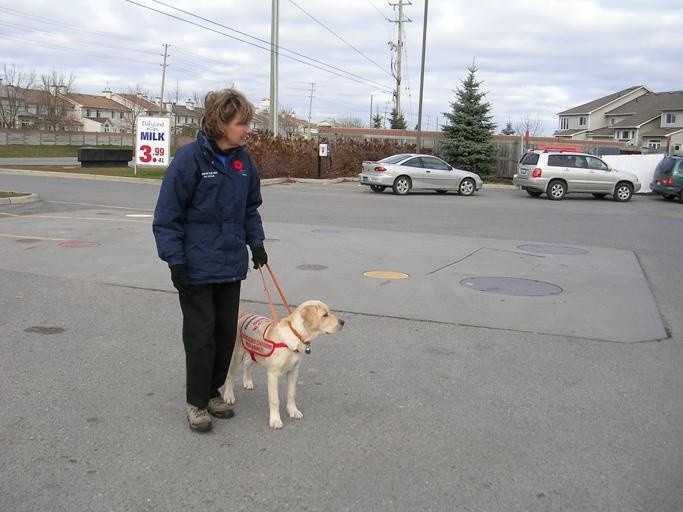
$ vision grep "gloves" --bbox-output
[169,263,192,292]
[251,247,267,270]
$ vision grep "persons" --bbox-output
[152,86,268,432]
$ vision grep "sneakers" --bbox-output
[207,395,235,419]
[186,402,213,432]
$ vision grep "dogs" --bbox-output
[223,300,344,429]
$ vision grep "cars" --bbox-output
[358,154,484,196]
[649,154,683,203]
[513,146,641,202]
